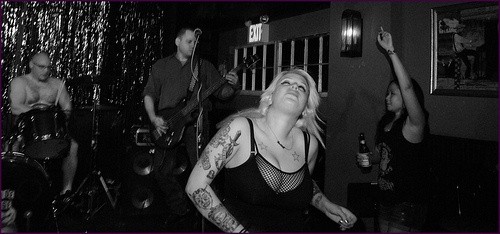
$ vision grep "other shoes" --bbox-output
[55,190,74,205]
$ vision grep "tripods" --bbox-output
[57,90,117,220]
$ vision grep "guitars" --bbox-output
[150,53,261,149]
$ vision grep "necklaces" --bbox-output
[387,119,396,131]
[265,116,294,151]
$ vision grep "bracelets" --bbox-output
[239,229,248,234]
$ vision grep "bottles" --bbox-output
[358,133,372,173]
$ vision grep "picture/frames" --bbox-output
[430,0,500,98]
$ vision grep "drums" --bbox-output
[19,105,71,160]
[1,151,59,232]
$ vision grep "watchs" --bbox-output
[387,49,395,55]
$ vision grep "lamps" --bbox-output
[340,9,363,58]
[244,13,269,45]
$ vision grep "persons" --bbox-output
[359,26,431,234]
[6,53,79,208]
[184,68,358,234]
[141,28,240,234]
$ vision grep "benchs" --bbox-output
[346,133,500,231]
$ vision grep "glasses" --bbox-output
[32,62,55,71]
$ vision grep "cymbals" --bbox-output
[80,106,120,111]
